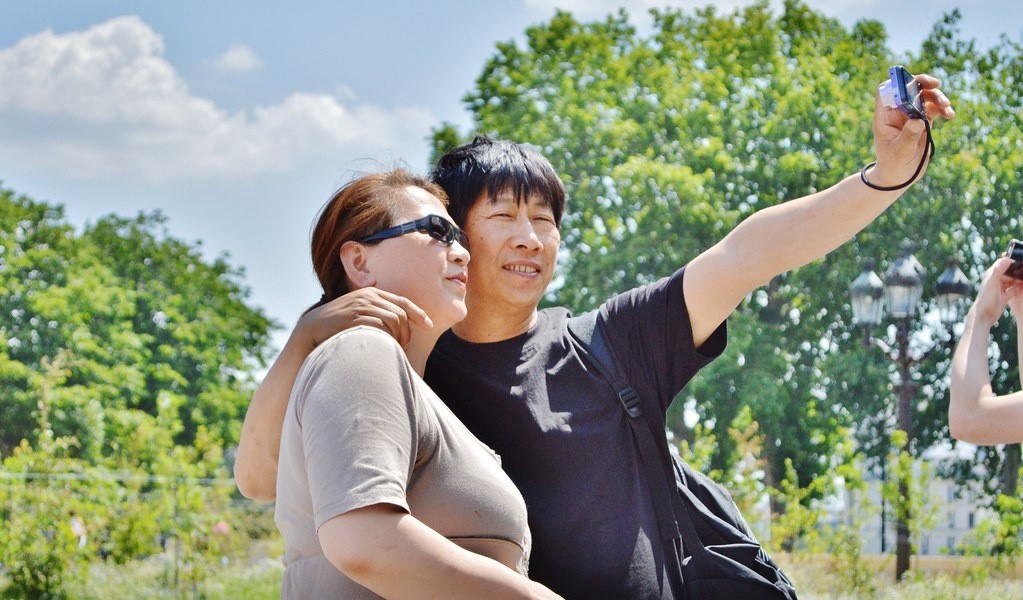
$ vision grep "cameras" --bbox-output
[1004,238,1023,281]
[879,65,927,119]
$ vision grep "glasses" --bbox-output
[358,215,471,252]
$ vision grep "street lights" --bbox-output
[846,251,972,584]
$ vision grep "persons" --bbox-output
[273,167,569,600]
[949,250,1023,446]
[232,73,953,600]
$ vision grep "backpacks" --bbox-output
[564,308,798,600]
